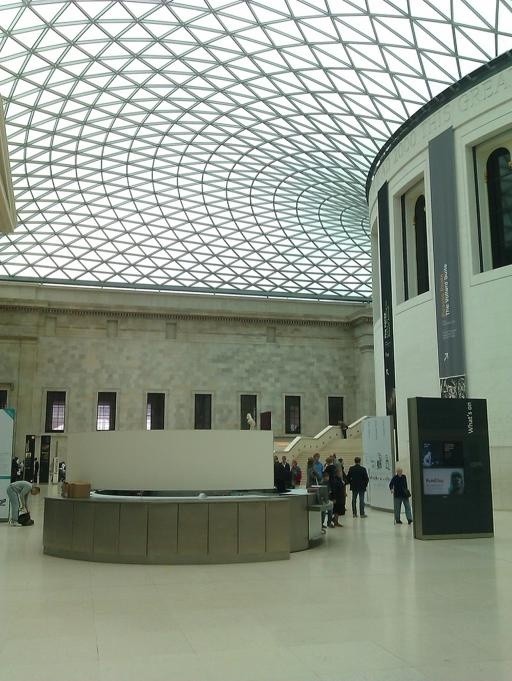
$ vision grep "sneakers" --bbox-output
[9,521,19,526]
[353,514,367,518]
[408,519,412,524]
[322,517,342,528]
[396,520,402,523]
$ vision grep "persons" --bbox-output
[11,457,21,483]
[57,461,67,484]
[389,467,412,525]
[272,454,371,529]
[7,480,41,528]
[339,420,348,439]
[34,458,40,484]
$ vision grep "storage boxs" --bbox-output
[62,481,91,498]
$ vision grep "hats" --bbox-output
[307,457,313,464]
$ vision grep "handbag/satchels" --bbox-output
[18,513,34,525]
[403,488,411,497]
